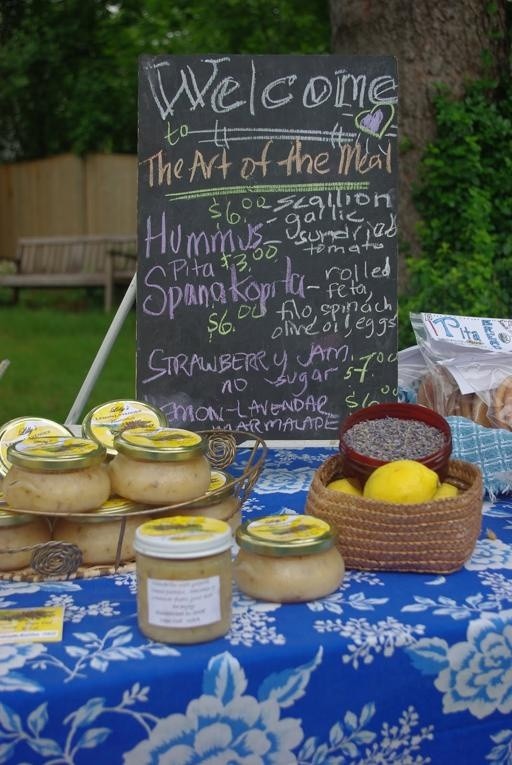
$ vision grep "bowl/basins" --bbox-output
[338,400,454,494]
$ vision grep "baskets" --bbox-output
[0,431,267,583]
[306,456,482,573]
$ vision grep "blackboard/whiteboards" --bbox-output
[135,53,400,440]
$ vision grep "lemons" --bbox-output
[326,459,459,506]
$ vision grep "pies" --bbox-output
[418,376,512,431]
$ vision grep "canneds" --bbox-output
[235,514,345,603]
[0,398,243,572]
[132,516,235,645]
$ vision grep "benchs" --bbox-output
[2,231,139,323]
